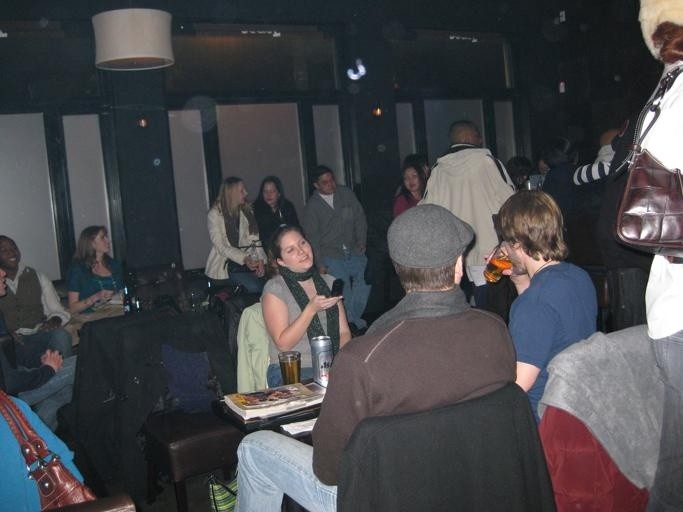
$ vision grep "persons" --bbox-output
[259,223,352,386]
[252,176,302,250]
[235,203,516,512]
[1,396,85,512]
[631,1,683,512]
[301,166,372,333]
[483,192,599,421]
[0,236,73,363]
[64,225,127,315]
[394,120,649,322]
[0,269,77,435]
[203,178,271,293]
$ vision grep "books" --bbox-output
[222,380,329,421]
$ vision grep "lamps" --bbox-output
[91,8,176,73]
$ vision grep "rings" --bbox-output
[103,294,108,298]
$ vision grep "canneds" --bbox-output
[311,336,335,387]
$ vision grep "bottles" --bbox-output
[119,276,144,318]
[187,278,216,316]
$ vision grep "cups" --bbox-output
[484,242,513,286]
[277,350,302,385]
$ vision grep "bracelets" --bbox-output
[88,296,95,305]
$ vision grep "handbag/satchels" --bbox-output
[222,245,273,299]
[204,467,239,512]
[0,387,96,512]
[610,66,683,256]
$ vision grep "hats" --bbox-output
[384,202,479,271]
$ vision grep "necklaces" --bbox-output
[91,265,118,295]
